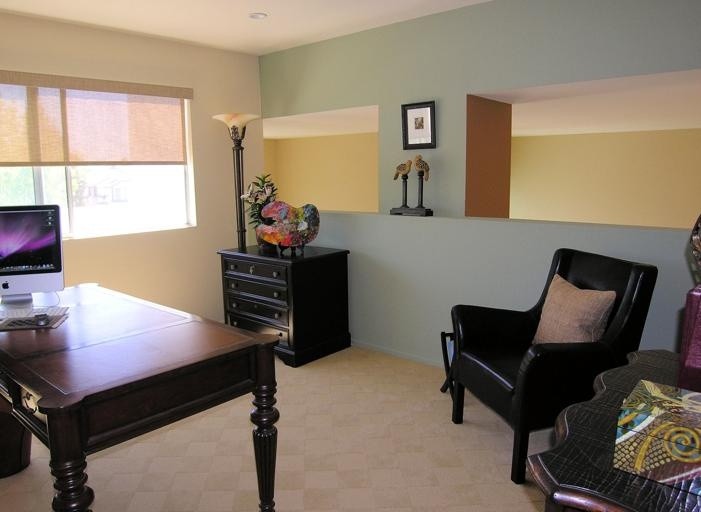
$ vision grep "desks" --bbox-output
[524,342,701,510]
[1,277,285,510]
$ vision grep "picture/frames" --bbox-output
[399,98,439,150]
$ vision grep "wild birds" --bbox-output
[393,160,412,180]
[415,154,429,181]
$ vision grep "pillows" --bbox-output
[532,273,618,345]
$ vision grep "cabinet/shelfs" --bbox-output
[216,239,353,368]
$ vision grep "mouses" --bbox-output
[34,314,49,326]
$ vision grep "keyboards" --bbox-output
[0,306,69,319]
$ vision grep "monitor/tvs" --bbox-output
[0,204,64,302]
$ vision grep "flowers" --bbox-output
[241,170,278,227]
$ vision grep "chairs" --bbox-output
[448,242,660,484]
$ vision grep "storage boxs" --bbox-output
[610,374,701,497]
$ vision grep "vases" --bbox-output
[254,225,278,254]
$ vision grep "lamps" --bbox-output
[210,110,270,255]
